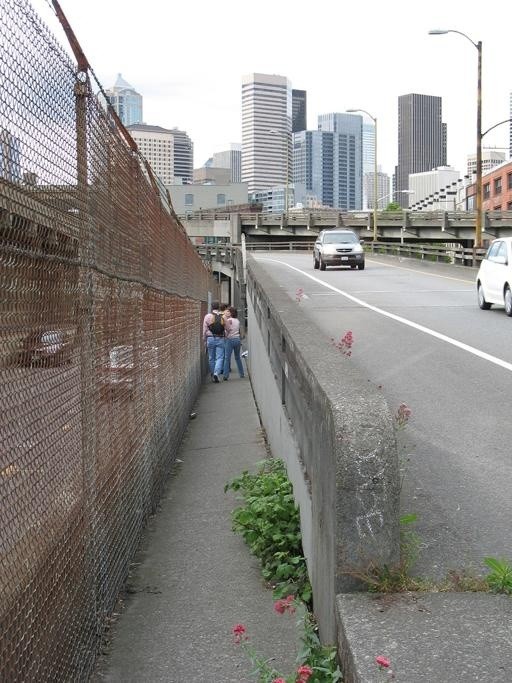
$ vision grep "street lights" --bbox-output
[346,108,415,242]
[270,129,289,219]
[428,27,484,250]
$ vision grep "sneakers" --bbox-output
[211,368,232,383]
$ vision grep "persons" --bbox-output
[202,300,245,382]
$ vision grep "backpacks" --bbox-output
[208,313,225,334]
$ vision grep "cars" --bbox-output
[18,327,73,368]
[312,228,367,271]
[97,343,161,397]
[475,237,511,317]
